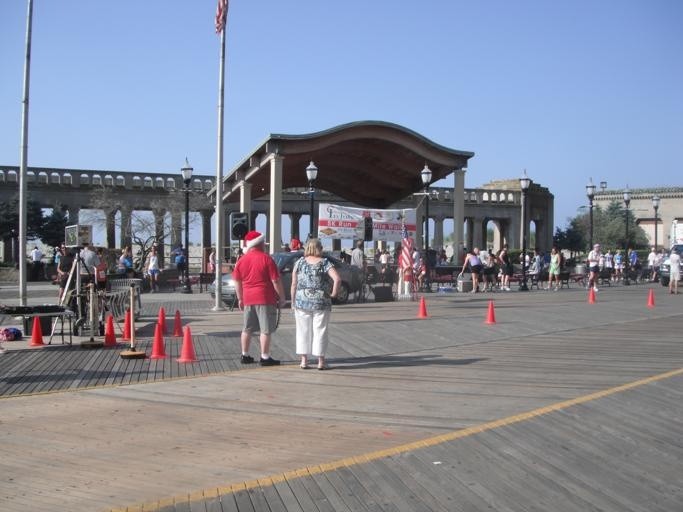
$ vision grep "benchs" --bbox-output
[433,272,625,293]
[0,309,74,350]
[198,273,228,293]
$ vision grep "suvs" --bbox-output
[658,244,683,287]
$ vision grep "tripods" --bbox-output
[47,248,124,344]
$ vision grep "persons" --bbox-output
[30,241,140,306]
[289,235,301,252]
[290,237,342,371]
[232,230,286,366]
[340,239,682,304]
[143,245,164,293]
[208,248,216,274]
[169,244,189,285]
[234,247,242,267]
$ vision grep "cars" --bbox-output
[206,250,365,305]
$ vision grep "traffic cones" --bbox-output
[415,295,430,318]
[101,315,120,348]
[587,286,596,304]
[28,315,47,347]
[120,309,133,342]
[483,300,497,324]
[149,305,198,364]
[646,289,656,307]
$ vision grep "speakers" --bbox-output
[229,212,249,240]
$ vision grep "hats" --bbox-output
[242,231,265,253]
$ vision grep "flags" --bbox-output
[401,214,414,282]
[215,0,228,35]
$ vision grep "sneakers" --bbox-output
[318,363,329,370]
[240,355,254,364]
[299,361,307,368]
[260,357,280,365]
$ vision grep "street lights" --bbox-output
[599,182,608,190]
[585,176,597,254]
[619,185,632,283]
[419,161,433,294]
[180,155,196,295]
[304,159,319,240]
[651,193,660,254]
[518,170,531,290]
